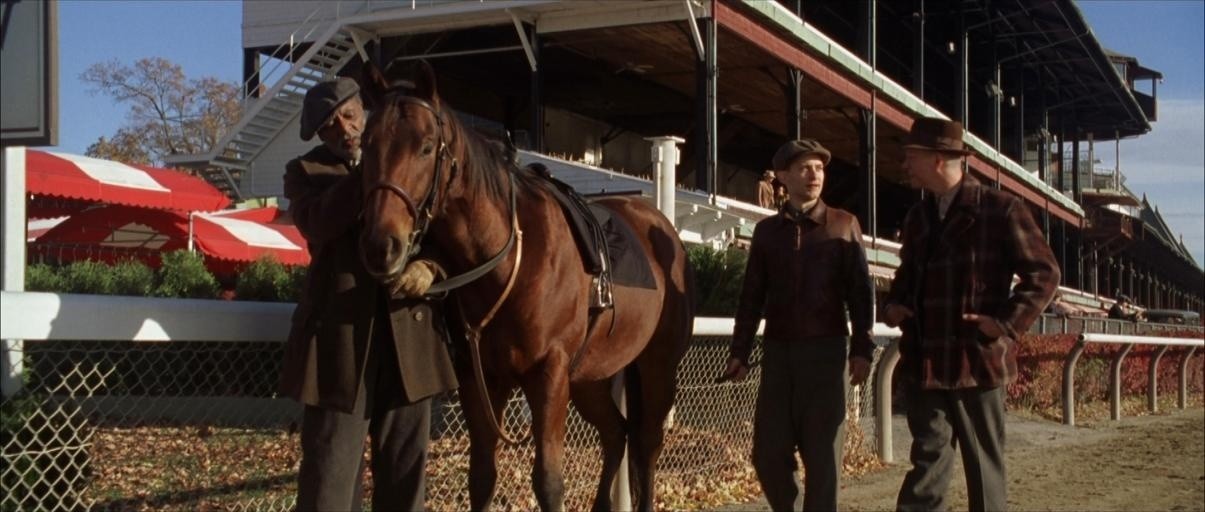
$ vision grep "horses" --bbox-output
[354,60,695,512]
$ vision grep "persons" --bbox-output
[880,115,1061,512]
[753,167,775,208]
[1107,295,1149,324]
[282,75,462,512]
[724,137,875,511]
[774,185,790,210]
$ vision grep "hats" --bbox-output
[301,76,360,140]
[772,139,831,171]
[900,117,971,156]
